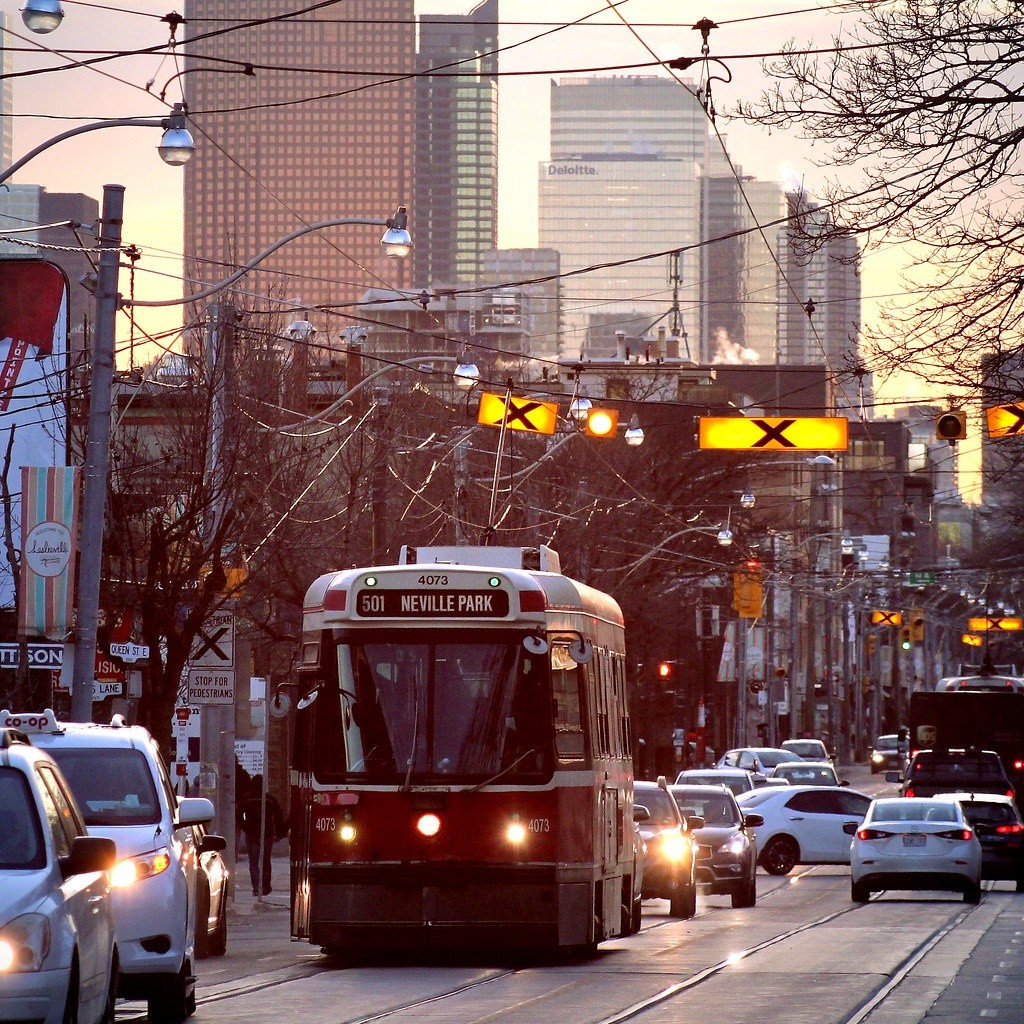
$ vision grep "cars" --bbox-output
[715,737,852,787]
[735,784,875,876]
[674,768,753,797]
[635,803,650,932]
[175,793,231,964]
[932,792,1024,893]
[868,734,909,775]
[844,799,983,904]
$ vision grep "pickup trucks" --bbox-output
[886,747,1016,803]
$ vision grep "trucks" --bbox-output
[898,691,1024,791]
[935,664,1024,692]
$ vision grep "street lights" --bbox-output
[731,528,890,767]
[212,345,480,537]
[451,413,645,547]
[68,206,416,725]
[374,383,593,569]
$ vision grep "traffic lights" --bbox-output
[936,411,967,440]
[911,608,924,640]
[659,664,670,680]
[586,407,618,439]
[899,626,914,652]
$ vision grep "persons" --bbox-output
[186,776,199,798]
[235,774,284,896]
[235,755,252,863]
[709,799,729,822]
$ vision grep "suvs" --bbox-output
[0,727,119,1024]
[0,711,215,1024]
[633,775,704,919]
[667,783,765,909]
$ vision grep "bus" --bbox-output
[268,544,636,964]
